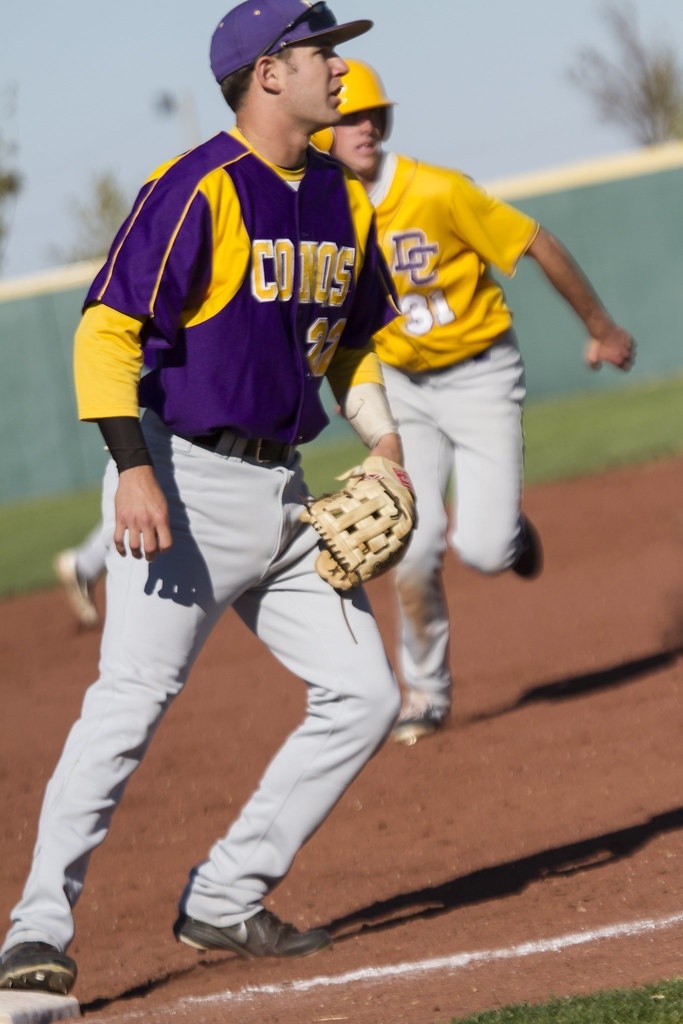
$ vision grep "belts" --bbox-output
[183,429,296,465]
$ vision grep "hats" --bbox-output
[210,0,374,81]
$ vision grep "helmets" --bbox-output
[309,59,394,154]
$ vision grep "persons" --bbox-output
[53,60,638,750]
[0,1,417,1024]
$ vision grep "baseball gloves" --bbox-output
[298,455,421,594]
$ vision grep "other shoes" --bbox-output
[508,514,544,581]
[392,700,452,739]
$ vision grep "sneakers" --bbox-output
[0,942,78,991]
[173,911,332,963]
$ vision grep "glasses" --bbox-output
[245,1,338,76]
